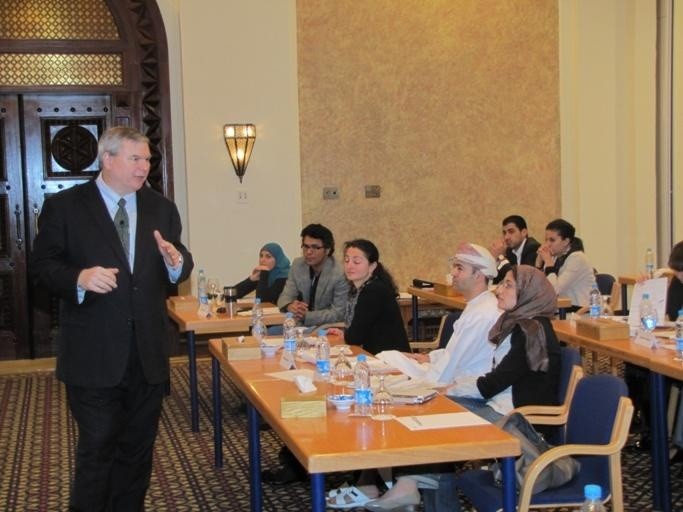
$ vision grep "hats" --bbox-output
[447,241,498,279]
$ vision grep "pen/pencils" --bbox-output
[236,308,252,312]
[640,317,658,350]
[641,272,648,282]
[376,370,400,375]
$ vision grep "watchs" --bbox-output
[178,252,184,269]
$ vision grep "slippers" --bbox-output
[326,486,382,509]
[324,482,353,498]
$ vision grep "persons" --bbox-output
[488,215,541,285]
[535,219,600,313]
[343,239,411,355]
[633,240,682,471]
[267,224,349,336]
[207,243,291,306]
[364,265,561,512]
[325,242,506,509]
[33,127,194,512]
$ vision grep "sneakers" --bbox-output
[262,464,306,485]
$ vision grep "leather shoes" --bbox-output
[669,444,683,465]
[364,491,422,512]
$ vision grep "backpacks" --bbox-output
[501,412,581,497]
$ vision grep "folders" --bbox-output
[413,279,433,288]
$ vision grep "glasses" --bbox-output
[301,244,324,250]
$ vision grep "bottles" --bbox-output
[251,298,395,423]
[195,270,237,318]
[588,281,602,317]
[638,293,682,361]
[642,245,657,279]
[578,484,608,512]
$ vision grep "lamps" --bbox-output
[223,122,256,183]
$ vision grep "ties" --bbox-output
[113,198,130,260]
